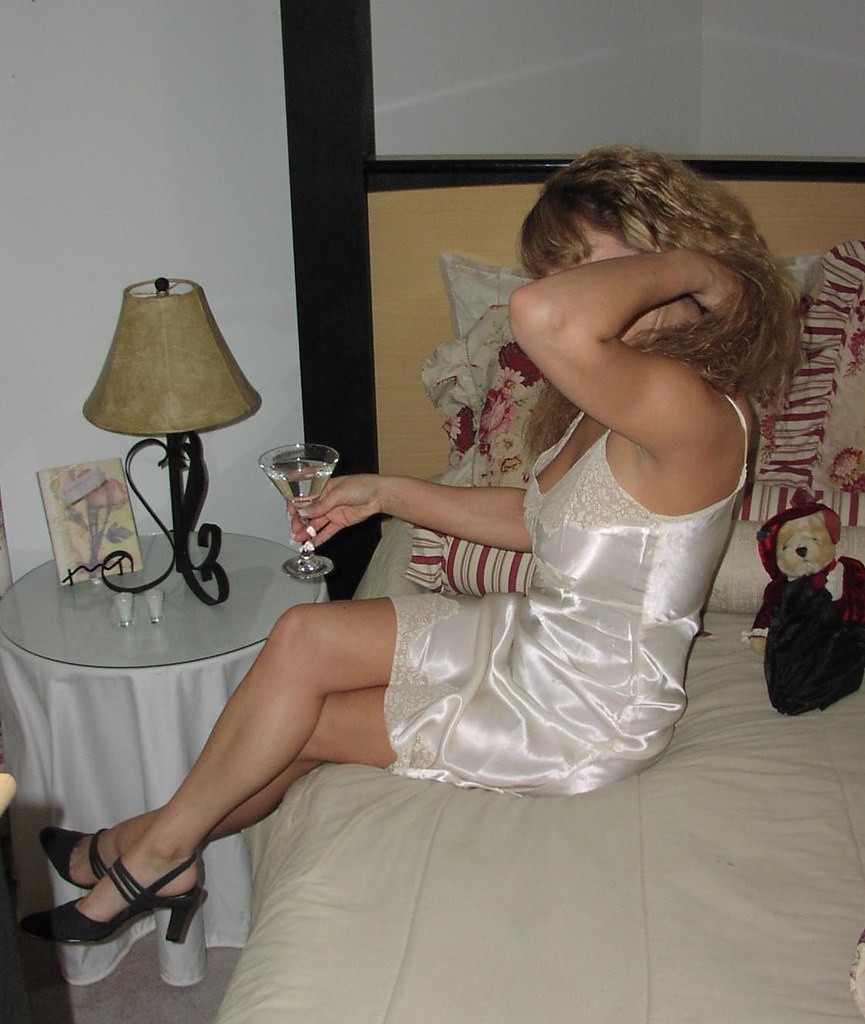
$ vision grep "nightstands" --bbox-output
[0,528,331,987]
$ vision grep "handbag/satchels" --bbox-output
[763,574,865,716]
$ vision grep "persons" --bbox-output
[16,144,800,943]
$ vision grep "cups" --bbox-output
[142,590,165,626]
[112,592,135,627]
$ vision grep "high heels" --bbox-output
[19,852,208,944]
[37,825,123,889]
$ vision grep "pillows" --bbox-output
[407,236,865,614]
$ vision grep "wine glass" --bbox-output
[257,442,340,581]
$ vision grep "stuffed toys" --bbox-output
[741,503,865,716]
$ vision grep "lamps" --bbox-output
[79,274,265,606]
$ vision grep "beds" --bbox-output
[212,158,864,1020]
